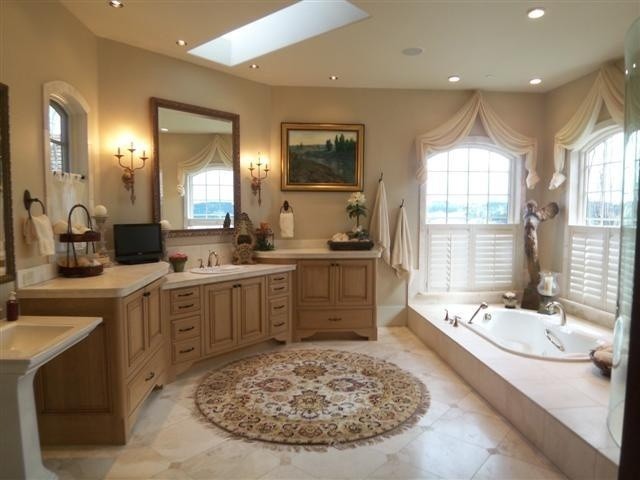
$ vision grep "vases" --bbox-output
[169,257,187,272]
[347,230,368,240]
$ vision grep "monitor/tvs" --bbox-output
[113,223,162,265]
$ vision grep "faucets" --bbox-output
[545,299,568,328]
[206,250,218,268]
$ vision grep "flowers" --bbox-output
[345,191,368,238]
[169,251,186,258]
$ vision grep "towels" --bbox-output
[23,214,55,257]
[369,182,391,266]
[279,213,295,239]
[58,256,100,268]
[331,232,348,242]
[52,218,91,235]
[392,207,411,280]
[594,344,613,364]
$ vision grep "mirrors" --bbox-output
[150,97,241,234]
[0,83,15,283]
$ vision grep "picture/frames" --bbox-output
[281,122,364,192]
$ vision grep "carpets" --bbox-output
[196,347,431,451]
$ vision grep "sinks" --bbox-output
[190,264,243,274]
[0,321,74,358]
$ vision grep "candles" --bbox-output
[544,277,552,296]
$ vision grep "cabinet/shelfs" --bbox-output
[170,285,201,365]
[268,270,289,336]
[296,258,373,330]
[204,276,266,354]
[18,275,168,445]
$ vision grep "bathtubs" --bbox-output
[444,298,617,362]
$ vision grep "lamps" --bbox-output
[114,131,148,205]
[90,203,115,268]
[249,152,270,203]
